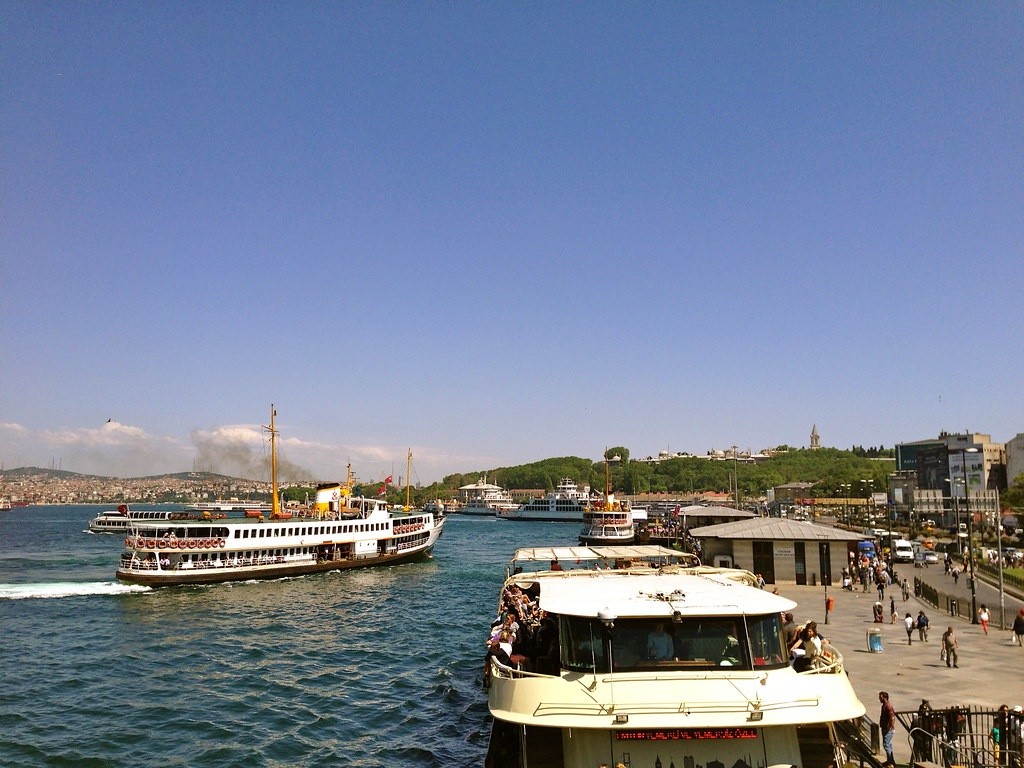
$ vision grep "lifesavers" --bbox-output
[333,492,337,496]
[188,541,197,548]
[158,540,167,549]
[394,523,425,534]
[217,537,223,543]
[125,538,128,545]
[212,540,218,547]
[129,540,134,548]
[198,540,205,548]
[593,518,628,525]
[331,496,338,500]
[179,540,187,549]
[219,540,225,547]
[170,539,178,548]
[205,539,212,548]
[147,540,156,548]
[137,539,145,548]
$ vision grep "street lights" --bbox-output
[946,478,965,553]
[731,445,741,509]
[836,484,852,523]
[861,479,874,529]
[962,447,979,625]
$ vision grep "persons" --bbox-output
[315,546,328,561]
[550,560,564,571]
[916,547,921,553]
[753,572,765,590]
[978,604,990,635]
[578,625,619,667]
[901,579,910,602]
[992,552,1019,569]
[993,705,1024,768]
[781,611,827,673]
[748,630,770,659]
[944,551,978,585]
[592,562,626,571]
[942,626,959,668]
[772,587,779,595]
[645,619,674,662]
[650,523,702,569]
[873,602,884,623]
[1013,608,1024,647]
[120,552,284,570]
[905,613,932,647]
[139,532,143,538]
[840,551,894,602]
[910,704,965,765]
[485,582,561,677]
[879,692,895,768]
[274,533,277,537]
[890,595,897,624]
[163,532,176,540]
[513,566,523,575]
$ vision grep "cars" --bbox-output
[925,552,939,563]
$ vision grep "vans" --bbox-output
[890,539,915,563]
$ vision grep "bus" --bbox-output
[872,529,902,560]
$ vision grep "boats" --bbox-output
[0,495,12,510]
[113,401,449,586]
[495,475,650,521]
[88,510,195,533]
[420,474,512,515]
[181,497,273,511]
[487,440,867,768]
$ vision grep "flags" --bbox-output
[376,482,387,494]
[116,505,128,515]
[385,475,393,483]
[674,505,680,520]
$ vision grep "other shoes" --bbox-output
[947,664,951,667]
[953,664,959,668]
[1019,645,1022,647]
[986,632,988,635]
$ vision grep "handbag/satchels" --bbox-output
[912,622,916,629]
[941,649,946,661]
[892,611,899,618]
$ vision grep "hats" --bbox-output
[1013,706,1022,712]
[919,610,925,615]
[1019,608,1024,616]
